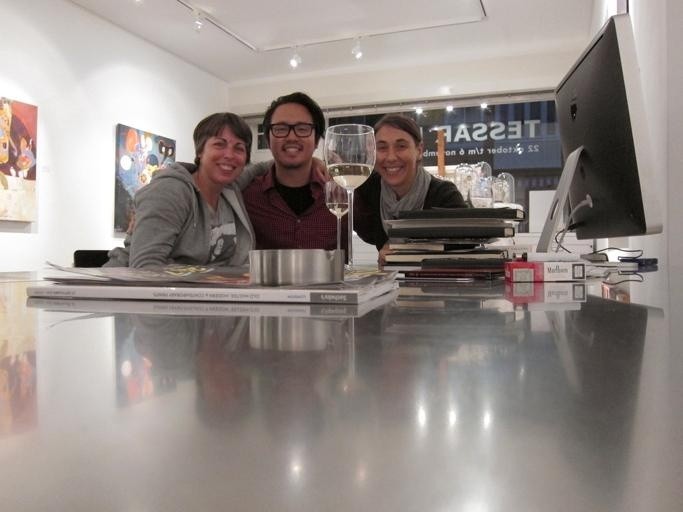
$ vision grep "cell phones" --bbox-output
[617,255,658,267]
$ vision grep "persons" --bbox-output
[124,91,354,263]
[327,114,479,270]
[100,112,333,279]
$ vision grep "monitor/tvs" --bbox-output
[536,12,664,279]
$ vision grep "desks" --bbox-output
[0,264,683,512]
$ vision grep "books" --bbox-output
[372,207,528,281]
[381,282,526,336]
[25,284,400,317]
[38,261,251,286]
[20,262,397,303]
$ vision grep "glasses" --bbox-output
[269,122,316,137]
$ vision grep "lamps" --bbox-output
[351,35,363,59]
[192,9,205,31]
[290,41,302,69]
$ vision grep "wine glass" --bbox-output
[324,122,377,273]
[325,180,351,249]
